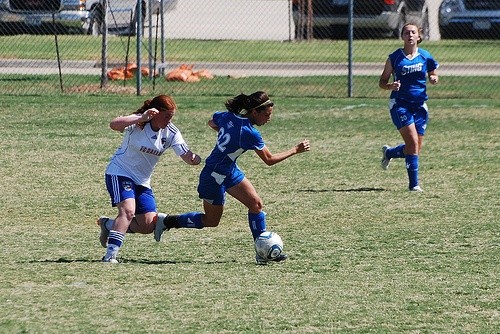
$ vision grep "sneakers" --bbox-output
[255,253,287,263]
[153,213,168,242]
[102,256,118,263]
[97,218,109,249]
[381,144,393,170]
[412,186,423,191]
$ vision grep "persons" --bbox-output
[87,0,104,36]
[378,23,439,191]
[131,0,146,37]
[154,91,310,264]
[98,95,201,263]
[387,0,408,40]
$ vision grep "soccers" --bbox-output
[254,230,285,260]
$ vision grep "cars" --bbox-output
[0,0,154,37]
[439,0,500,39]
[292,0,430,41]
